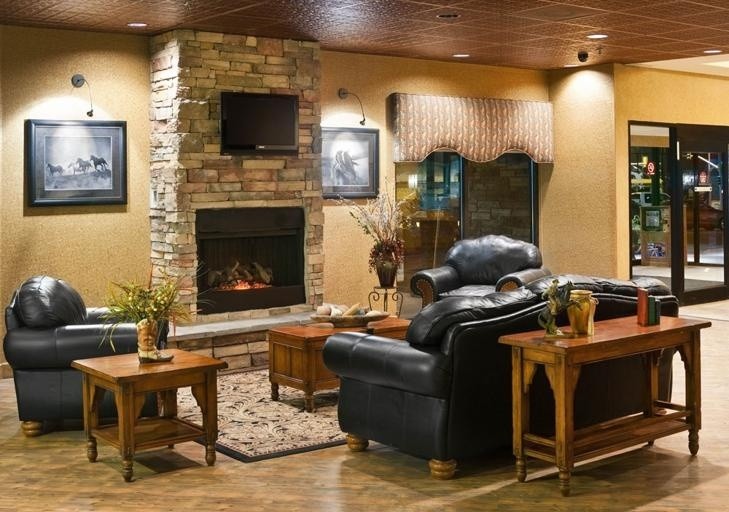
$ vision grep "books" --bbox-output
[648,296,655,325]
[655,300,660,324]
[637,288,649,326]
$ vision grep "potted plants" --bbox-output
[94,258,217,358]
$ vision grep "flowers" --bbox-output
[325,177,421,274]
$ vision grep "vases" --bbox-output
[567,288,600,336]
[376,255,400,287]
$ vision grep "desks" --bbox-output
[268,313,413,413]
[497,312,713,498]
[68,349,229,487]
[366,282,405,318]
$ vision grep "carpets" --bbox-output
[168,366,351,464]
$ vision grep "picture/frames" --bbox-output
[319,125,382,202]
[21,116,130,212]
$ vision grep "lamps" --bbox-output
[337,85,368,128]
[70,73,97,119]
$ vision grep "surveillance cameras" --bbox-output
[578,54,588,62]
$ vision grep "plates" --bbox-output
[310,311,392,328]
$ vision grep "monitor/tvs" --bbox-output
[221,91,299,156]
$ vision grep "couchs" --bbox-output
[409,230,551,310]
[321,271,681,481]
[3,271,170,443]
